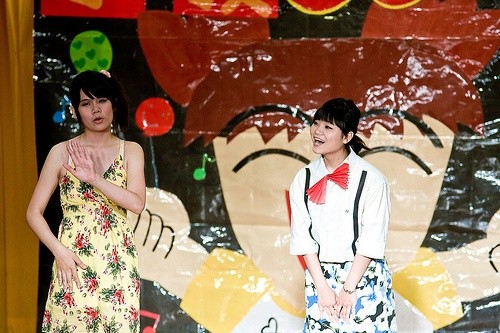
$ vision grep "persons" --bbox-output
[289,97,397,333]
[25,69,146,333]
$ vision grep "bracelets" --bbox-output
[343,285,356,294]
